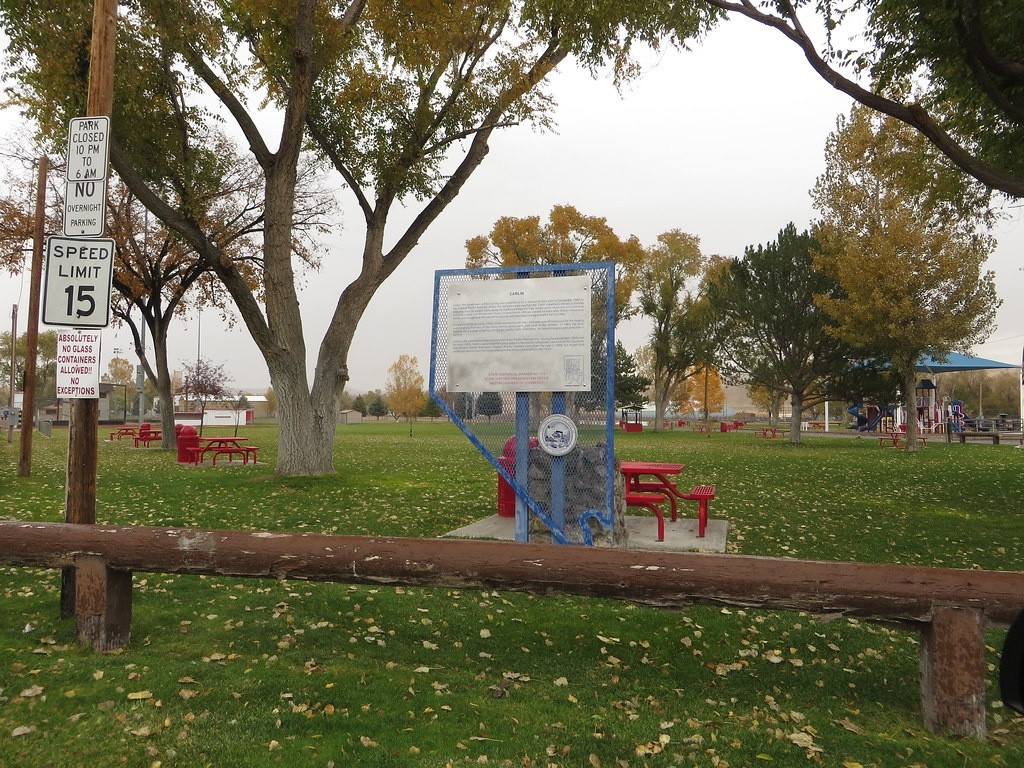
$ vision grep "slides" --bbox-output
[962,412,989,432]
[859,406,894,432]
[847,402,866,430]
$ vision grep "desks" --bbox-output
[760,427,777,438]
[808,420,842,431]
[116,427,163,435]
[199,436,248,462]
[619,460,686,521]
[886,432,906,439]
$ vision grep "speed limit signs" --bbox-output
[41,235,115,328]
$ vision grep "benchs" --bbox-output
[689,485,717,537]
[661,419,746,432]
[624,493,666,541]
[754,430,787,439]
[878,436,928,449]
[110,432,163,448]
[628,482,678,522]
[185,445,260,466]
[956,432,999,445]
